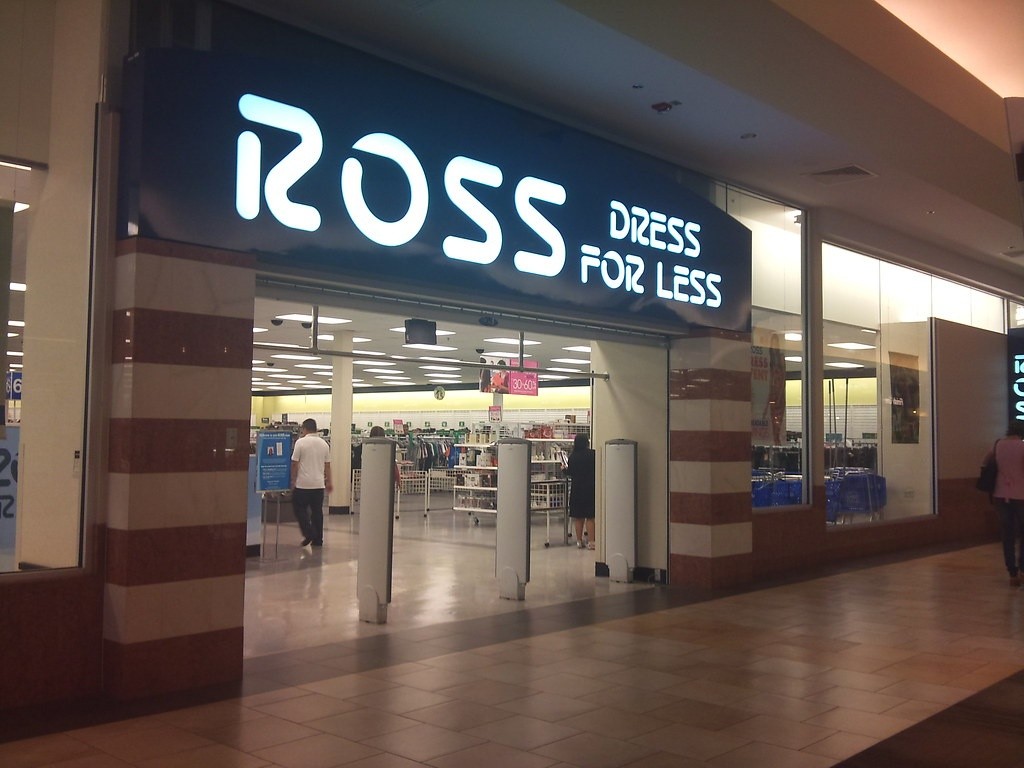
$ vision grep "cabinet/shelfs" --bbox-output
[453,420,590,526]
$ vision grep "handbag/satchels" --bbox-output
[975,439,998,496]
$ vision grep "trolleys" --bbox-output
[751,465,887,527]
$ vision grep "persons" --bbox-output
[400,425,410,461]
[289,418,333,546]
[268,449,273,455]
[980,419,1024,587]
[479,357,491,393]
[491,360,509,394]
[369,426,401,492]
[560,433,596,549]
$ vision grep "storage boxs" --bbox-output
[480,474,497,487]
[459,454,466,465]
[565,415,576,424]
[466,450,481,466]
[481,453,492,466]
[464,473,483,487]
[476,455,487,467]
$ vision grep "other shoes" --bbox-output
[1010,576,1019,586]
[302,534,314,546]
[577,540,585,548]
[310,542,322,546]
[1018,572,1024,582]
[587,543,595,550]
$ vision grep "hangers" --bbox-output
[420,437,454,447]
[753,438,877,456]
